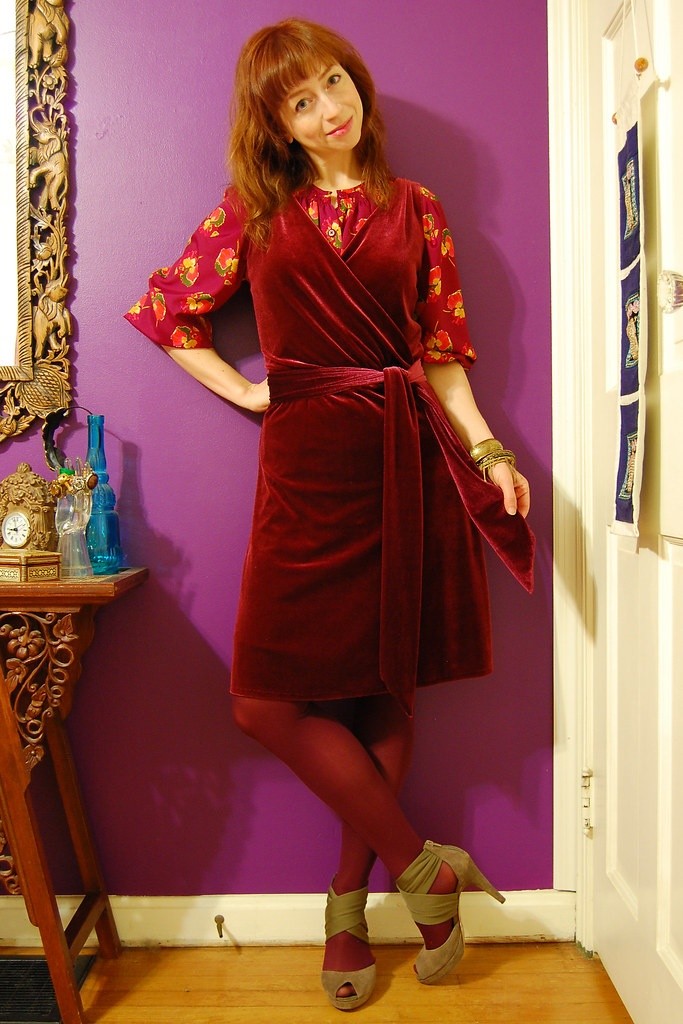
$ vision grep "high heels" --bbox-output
[319,874,377,1011]
[393,839,505,984]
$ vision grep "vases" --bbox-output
[81,414,130,574]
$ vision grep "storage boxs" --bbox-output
[0,548,62,581]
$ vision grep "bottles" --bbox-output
[83,414,123,572]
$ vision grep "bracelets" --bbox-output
[467,438,516,471]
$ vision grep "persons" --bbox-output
[121,19,535,1011]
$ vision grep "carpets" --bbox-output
[0,952,97,1024]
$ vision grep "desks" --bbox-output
[0,566,154,1024]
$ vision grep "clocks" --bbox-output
[0,463,71,550]
